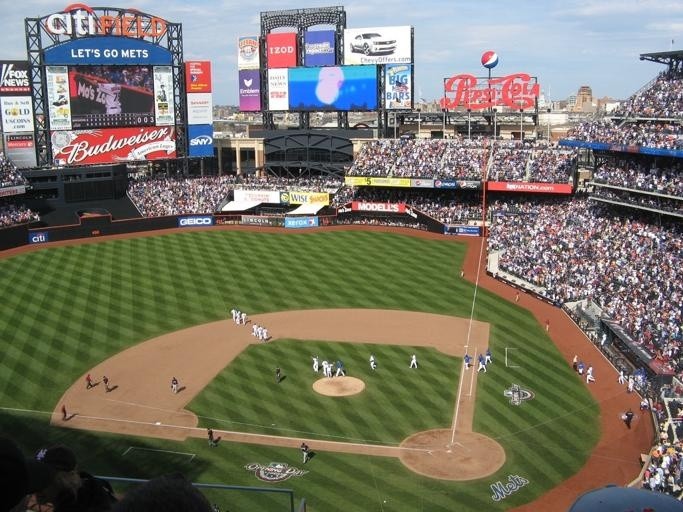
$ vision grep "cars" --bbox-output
[347,31,398,56]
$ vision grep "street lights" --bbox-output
[441,107,446,138]
[392,108,397,139]
[545,107,551,140]
[416,107,421,138]
[466,108,471,139]
[518,107,524,139]
[492,107,496,139]
